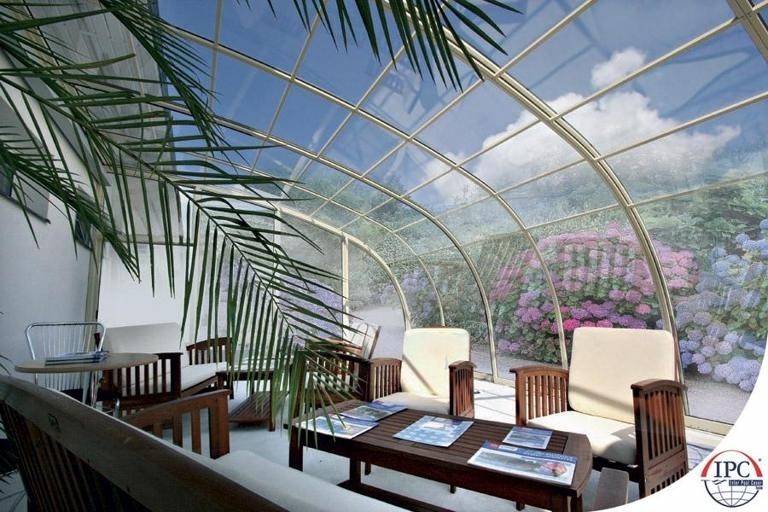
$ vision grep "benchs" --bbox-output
[94,321,236,429]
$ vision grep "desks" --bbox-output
[16,351,158,417]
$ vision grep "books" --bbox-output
[500,425,552,451]
[465,438,579,486]
[391,414,477,449]
[293,412,379,440]
[45,358,106,365]
[44,350,108,360]
[339,399,409,421]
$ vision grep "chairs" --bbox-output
[289,319,687,510]
[1,373,408,511]
